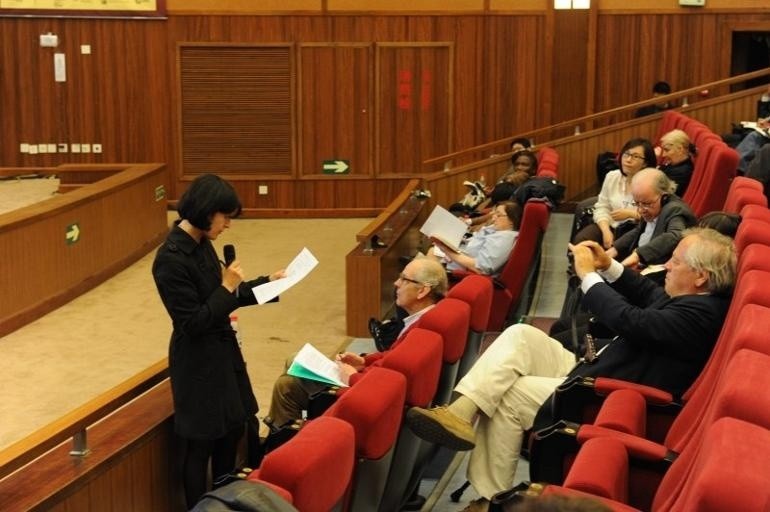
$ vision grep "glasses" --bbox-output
[399,273,418,283]
[623,151,646,161]
[630,199,655,208]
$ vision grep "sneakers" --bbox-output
[406,404,475,451]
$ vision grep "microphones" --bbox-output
[223,244,240,304]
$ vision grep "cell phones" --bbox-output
[660,192,668,207]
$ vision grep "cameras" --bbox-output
[567,249,574,275]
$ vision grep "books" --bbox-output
[428,233,460,253]
[285,342,352,389]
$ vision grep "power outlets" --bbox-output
[19,142,103,155]
[258,184,269,196]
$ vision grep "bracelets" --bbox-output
[465,219,472,226]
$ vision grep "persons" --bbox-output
[429,137,537,286]
[633,81,673,119]
[151,174,289,512]
[547,128,743,354]
[403,226,739,511]
[723,116,769,196]
[259,255,449,445]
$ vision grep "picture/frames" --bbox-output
[0,1,171,21]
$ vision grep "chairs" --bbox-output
[411,108,770,327]
[187,327,770,512]
